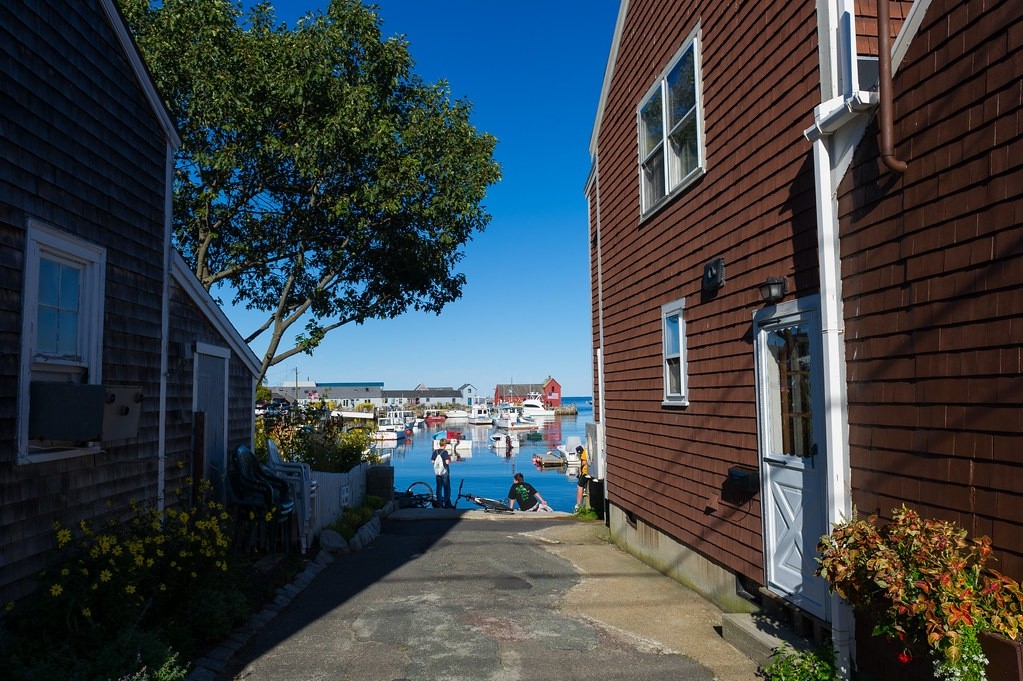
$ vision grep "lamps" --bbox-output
[758,276,787,303]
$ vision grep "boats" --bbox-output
[490,448,519,458]
[468,406,492,423]
[386,411,416,430]
[378,418,407,432]
[432,430,472,450]
[447,450,472,462]
[489,433,520,448]
[495,405,538,427]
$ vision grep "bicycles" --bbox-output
[393,481,444,509]
[453,478,515,513]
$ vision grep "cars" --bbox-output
[281,407,291,415]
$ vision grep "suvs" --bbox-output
[280,404,290,409]
[255,406,281,418]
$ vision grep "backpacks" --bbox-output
[433,450,447,476]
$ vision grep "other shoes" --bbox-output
[448,505,455,509]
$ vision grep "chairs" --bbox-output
[236,440,314,559]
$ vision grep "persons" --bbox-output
[508,473,553,512]
[576,446,589,505]
[431,438,453,507]
